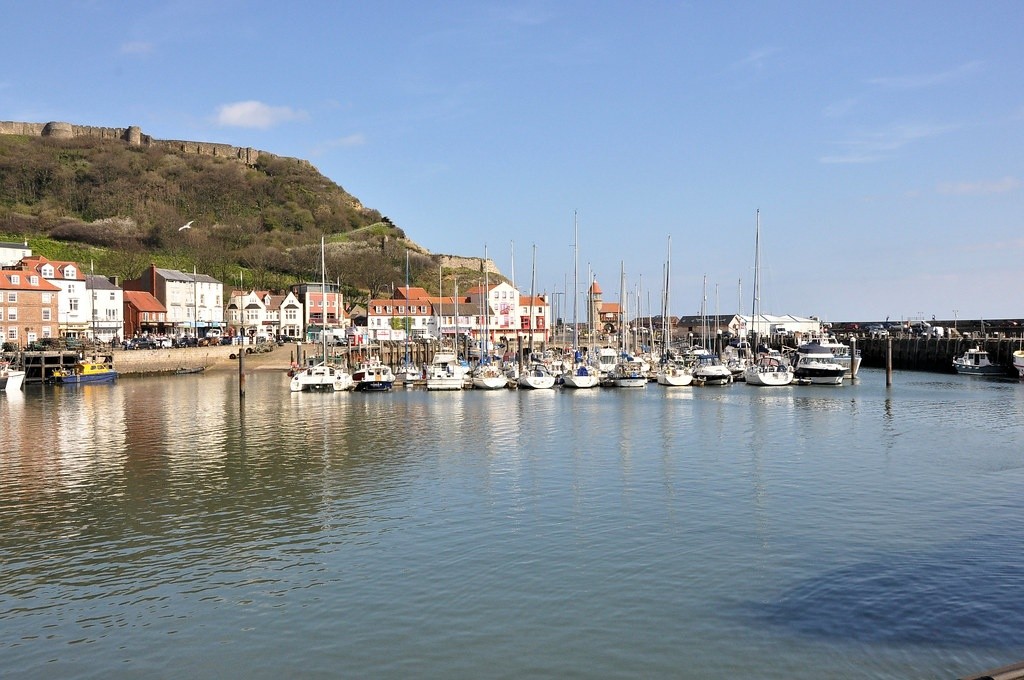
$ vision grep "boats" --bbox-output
[950,346,1009,377]
[49,348,120,385]
[0,360,27,390]
[1012,333,1024,378]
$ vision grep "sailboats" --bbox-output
[292,206,868,396]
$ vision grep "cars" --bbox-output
[125,329,233,350]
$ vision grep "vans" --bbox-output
[869,320,960,338]
